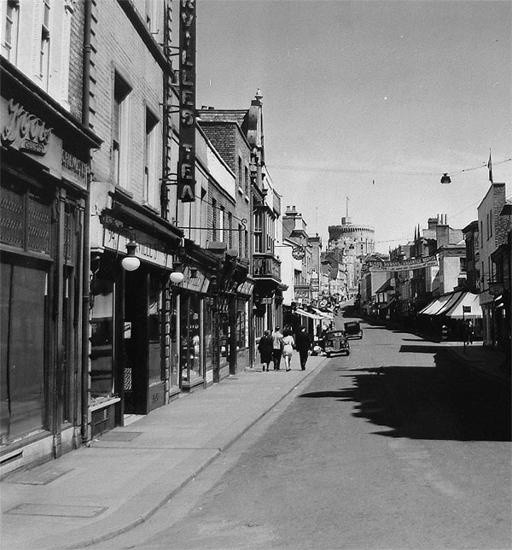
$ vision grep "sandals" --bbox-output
[262,365,269,371]
[286,368,292,372]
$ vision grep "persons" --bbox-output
[272,325,284,370]
[296,326,313,370]
[258,329,273,371]
[316,324,322,338]
[466,320,474,345]
[282,329,295,371]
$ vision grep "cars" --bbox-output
[324,320,363,358]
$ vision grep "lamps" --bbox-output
[111,227,142,273]
[162,246,184,283]
